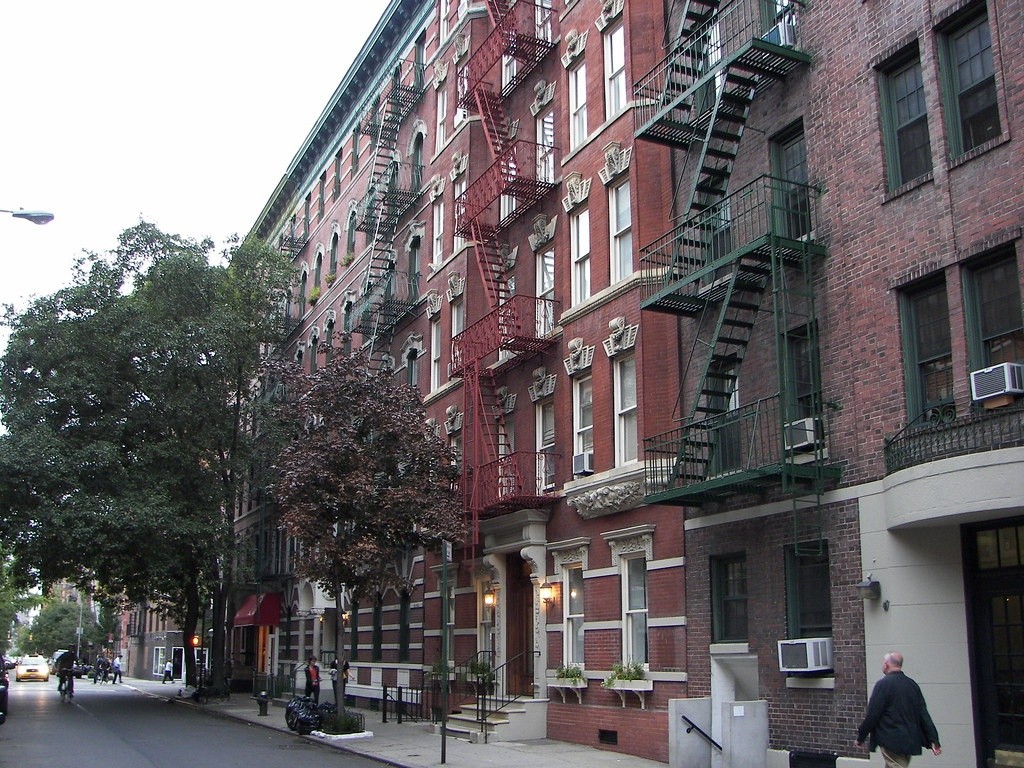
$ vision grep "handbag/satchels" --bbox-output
[112,666,120,673]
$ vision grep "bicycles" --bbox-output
[98,668,110,685]
[56,667,76,704]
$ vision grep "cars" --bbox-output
[0,649,93,726]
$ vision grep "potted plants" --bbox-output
[424,662,457,681]
[307,286,321,306]
[546,664,589,690]
[599,654,653,692]
[465,653,493,682]
[324,273,336,287]
[340,252,356,270]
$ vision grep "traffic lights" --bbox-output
[193,636,200,645]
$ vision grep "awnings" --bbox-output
[233,592,281,627]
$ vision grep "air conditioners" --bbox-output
[784,416,824,451]
[760,22,794,60]
[777,636,832,674]
[572,450,593,475]
[970,360,1024,403]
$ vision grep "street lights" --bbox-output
[76,602,83,660]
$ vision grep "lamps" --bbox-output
[482,582,497,609]
[341,611,350,621]
[319,615,327,624]
[539,574,556,606]
[855,573,884,600]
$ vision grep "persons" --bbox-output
[93,654,124,685]
[329,650,356,707]
[56,645,80,698]
[305,656,322,705]
[162,659,176,684]
[853,651,943,768]
